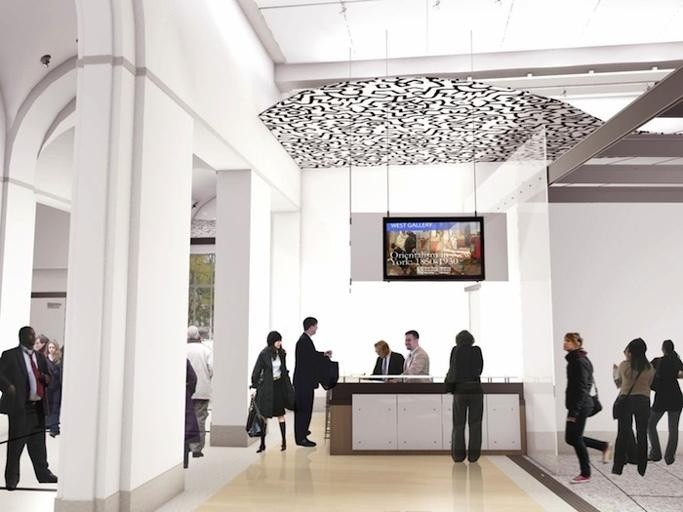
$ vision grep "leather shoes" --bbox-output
[305,429,311,435]
[38,474,57,483]
[6,479,15,489]
[295,438,316,447]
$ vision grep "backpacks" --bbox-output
[246,399,265,437]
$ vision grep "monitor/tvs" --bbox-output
[383,217,485,281]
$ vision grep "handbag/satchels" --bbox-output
[587,393,602,418]
[611,396,628,420]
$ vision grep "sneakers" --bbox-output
[603,440,611,464]
[569,473,593,483]
[48,430,59,437]
[192,452,203,457]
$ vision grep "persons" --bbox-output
[561,332,614,484]
[251,330,292,452]
[392,243,409,272]
[445,329,484,462]
[183,359,198,469]
[612,337,657,477]
[372,340,405,382]
[647,339,683,465]
[292,317,332,447]
[0,326,63,491]
[387,330,429,382]
[404,231,416,252]
[187,325,214,457]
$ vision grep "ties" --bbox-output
[23,349,43,398]
[382,357,386,380]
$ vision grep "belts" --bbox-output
[23,400,42,405]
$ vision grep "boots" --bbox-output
[278,422,286,452]
[256,424,265,453]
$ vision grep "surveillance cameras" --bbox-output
[41,55,50,67]
